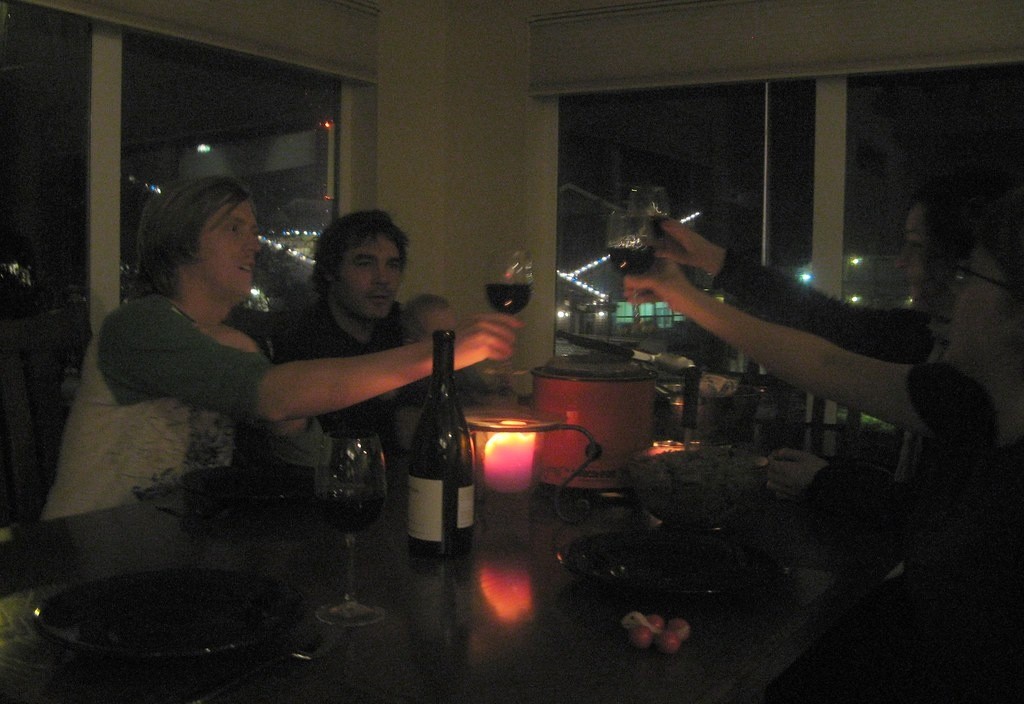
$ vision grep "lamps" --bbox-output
[464,410,600,522]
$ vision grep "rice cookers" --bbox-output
[526,352,655,492]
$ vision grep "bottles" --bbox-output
[407,331,475,564]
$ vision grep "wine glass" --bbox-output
[481,252,535,378]
[605,210,665,335]
[305,436,389,629]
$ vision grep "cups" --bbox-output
[626,184,670,235]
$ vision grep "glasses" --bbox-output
[952,260,1012,298]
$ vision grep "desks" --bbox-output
[0,451,905,704]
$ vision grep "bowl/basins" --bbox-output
[570,532,746,603]
[660,382,760,427]
[33,567,303,662]
[181,461,316,516]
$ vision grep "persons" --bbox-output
[624,177,1024,704]
[274,206,524,449]
[38,173,524,525]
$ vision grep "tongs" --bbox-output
[556,330,695,372]
